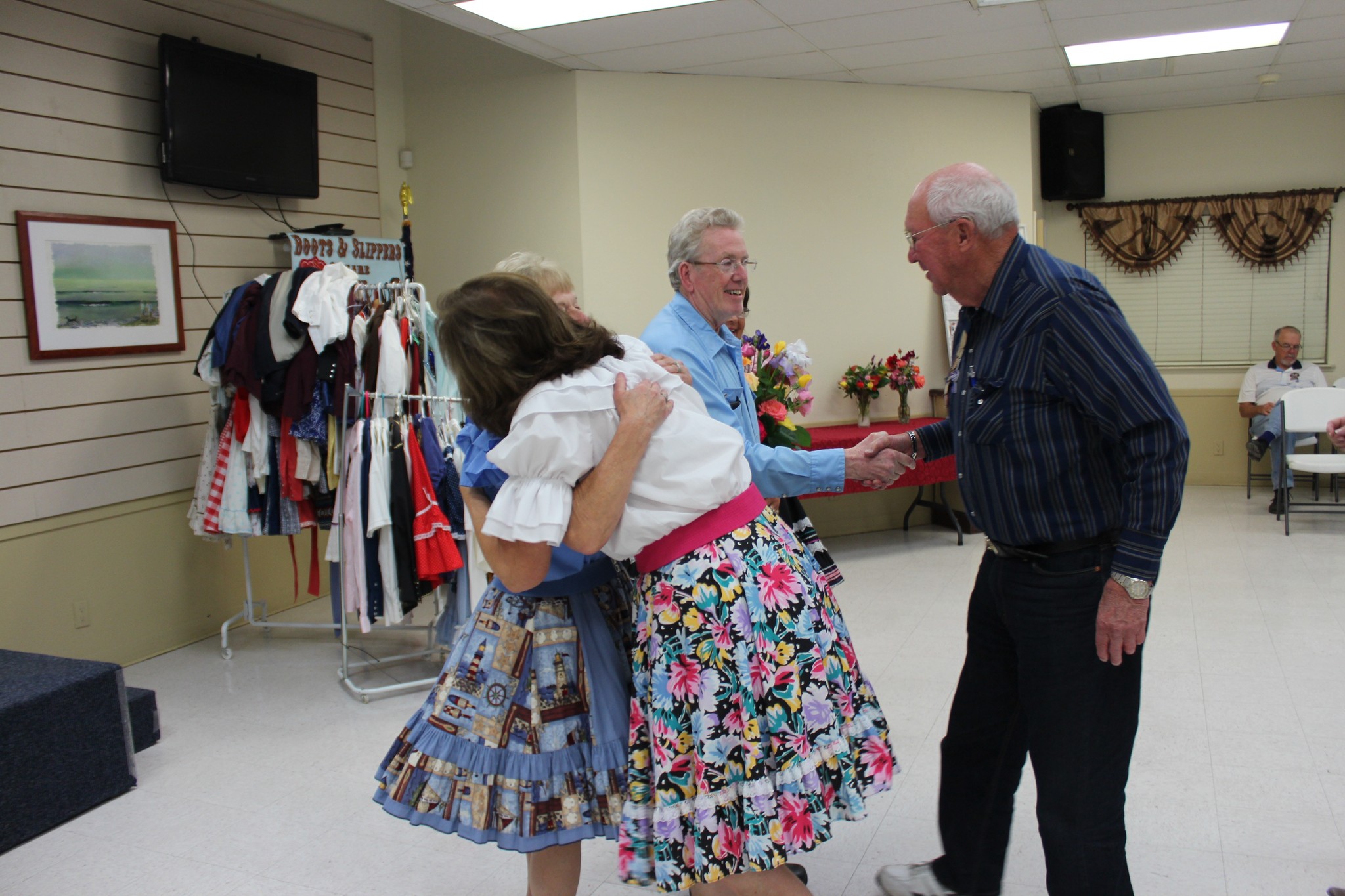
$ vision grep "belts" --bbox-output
[984,535,1109,565]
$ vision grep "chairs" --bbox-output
[1246,402,1319,502]
[1272,386,1345,538]
[1329,378,1345,502]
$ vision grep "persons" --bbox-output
[859,160,1193,896]
[369,249,697,896]
[636,206,916,514]
[434,271,902,896]
[724,285,752,339]
[1325,416,1345,449]
[1237,324,1329,515]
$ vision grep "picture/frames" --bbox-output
[13,208,187,362]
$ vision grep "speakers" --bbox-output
[1039,109,1104,201]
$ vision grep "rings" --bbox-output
[661,393,668,404]
[671,362,682,373]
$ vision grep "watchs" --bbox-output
[1111,570,1155,600]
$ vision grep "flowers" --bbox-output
[743,329,816,452]
[836,355,891,400]
[883,347,925,393]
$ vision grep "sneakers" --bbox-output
[1246,438,1268,461]
[1269,489,1292,514]
[877,861,963,896]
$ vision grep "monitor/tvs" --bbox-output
[159,34,320,199]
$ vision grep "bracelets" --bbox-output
[905,431,919,460]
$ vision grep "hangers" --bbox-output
[347,276,460,438]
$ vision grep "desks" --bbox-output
[788,417,964,547]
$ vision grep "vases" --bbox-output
[897,388,910,424]
[854,394,871,428]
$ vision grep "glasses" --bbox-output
[1274,340,1302,351]
[686,258,757,275]
[737,307,750,318]
